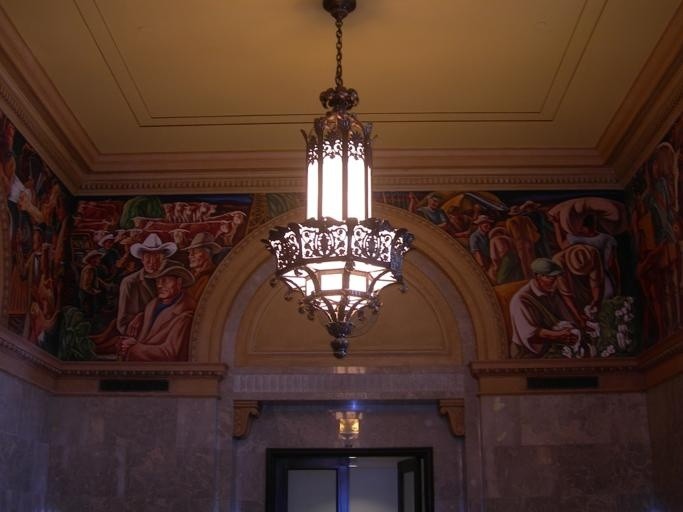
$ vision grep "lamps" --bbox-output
[261,1,415,362]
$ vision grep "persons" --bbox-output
[0,114,243,364]
[409,191,623,358]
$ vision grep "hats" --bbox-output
[564,245,593,275]
[145,260,195,287]
[180,232,222,254]
[130,233,177,259]
[99,234,117,246]
[426,193,443,201]
[509,201,541,216]
[474,216,493,223]
[531,258,564,276]
[82,250,102,264]
